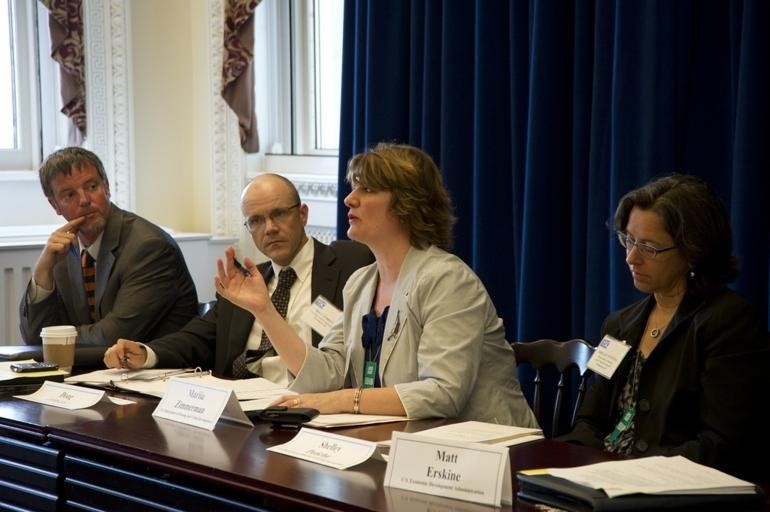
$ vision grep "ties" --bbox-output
[233,269,298,379]
[81,251,97,322]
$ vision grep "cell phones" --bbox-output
[11,360,60,373]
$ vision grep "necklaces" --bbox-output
[651,305,672,339]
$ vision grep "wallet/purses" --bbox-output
[261,405,320,423]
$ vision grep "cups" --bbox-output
[40,326,77,374]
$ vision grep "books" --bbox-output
[1,347,768,511]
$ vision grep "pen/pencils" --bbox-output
[232,256,253,277]
[119,357,129,364]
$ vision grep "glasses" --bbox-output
[244,203,300,232]
[615,230,679,260]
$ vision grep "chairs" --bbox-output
[506,340,597,442]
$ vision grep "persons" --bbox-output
[211,143,546,436]
[17,146,200,371]
[551,172,769,479]
[103,174,373,394]
